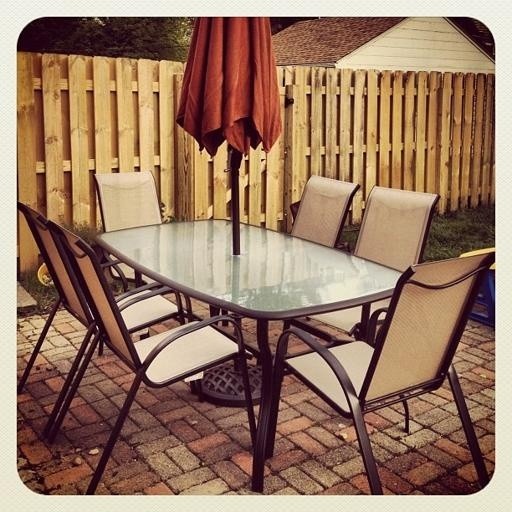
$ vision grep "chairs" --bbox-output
[305,186,440,340]
[49,220,256,492]
[92,170,163,287]
[19,202,186,436]
[290,175,361,321]
[267,249,495,492]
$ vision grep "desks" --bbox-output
[95,218,410,493]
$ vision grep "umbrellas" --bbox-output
[176,16,285,255]
